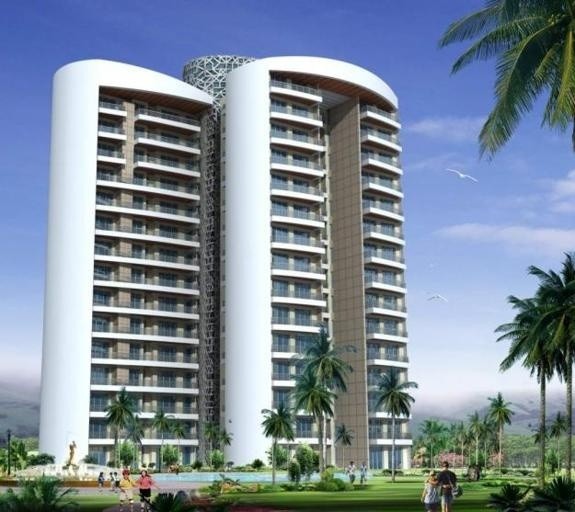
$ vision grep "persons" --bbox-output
[359,462,367,484]
[344,460,357,484]
[132,470,162,512]
[421,461,480,512]
[114,471,125,493]
[118,469,139,512]
[170,464,174,473]
[175,463,180,475]
[97,471,105,492]
[107,472,115,491]
